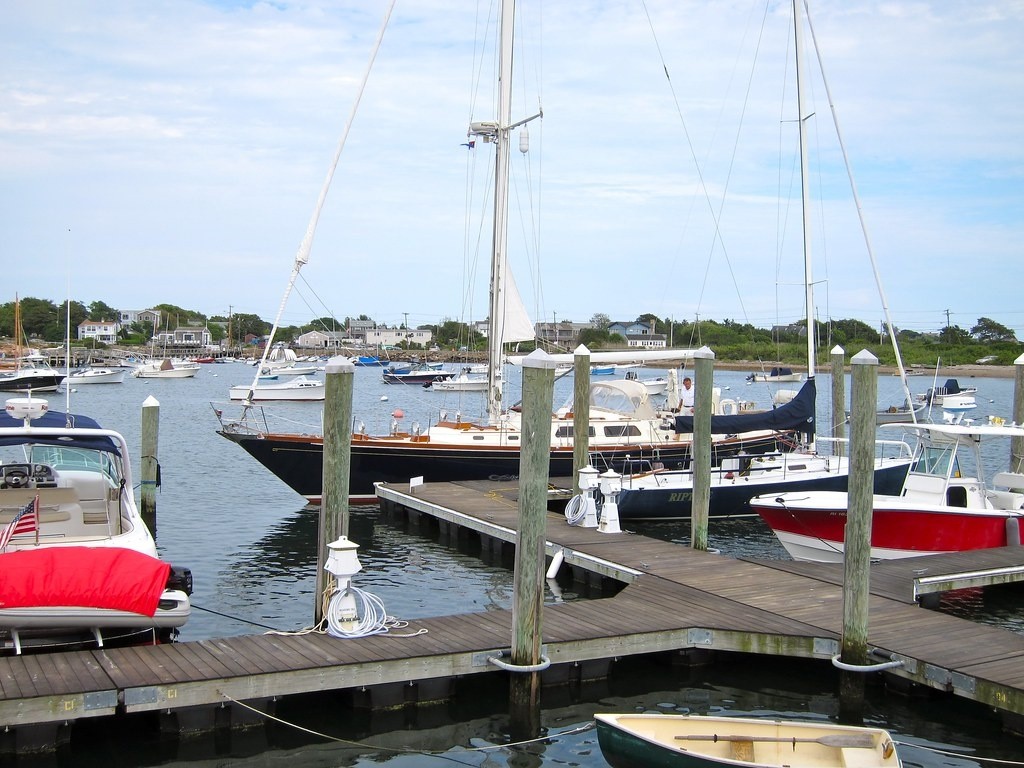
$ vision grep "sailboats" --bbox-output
[591,0,939,521]
[208,0,800,508]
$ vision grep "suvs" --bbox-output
[975,355,1000,365]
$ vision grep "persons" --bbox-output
[672,378,695,441]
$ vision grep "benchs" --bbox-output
[839,747,881,768]
[0,486,84,537]
[730,735,755,762]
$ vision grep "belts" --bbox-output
[683,405,694,407]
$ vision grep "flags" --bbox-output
[0,498,39,549]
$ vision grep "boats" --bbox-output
[593,713,903,768]
[748,411,1024,565]
[0,347,494,403]
[844,403,927,423]
[916,378,979,405]
[0,394,193,628]
